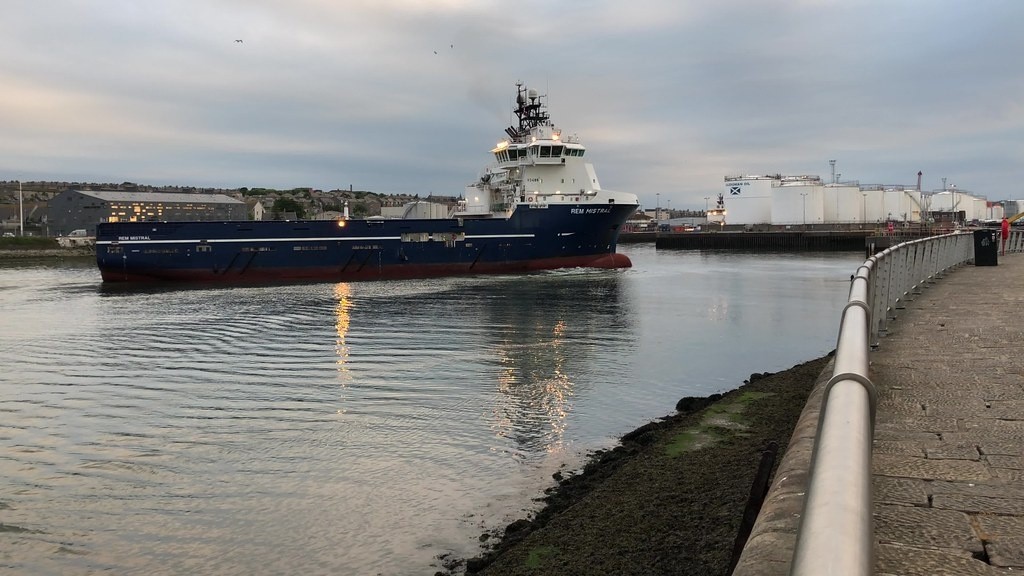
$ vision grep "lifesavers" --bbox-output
[528,197,533,202]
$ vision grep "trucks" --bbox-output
[67,229,88,237]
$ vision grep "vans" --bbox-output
[659,224,671,232]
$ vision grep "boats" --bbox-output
[89,78,642,290]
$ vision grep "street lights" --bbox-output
[950,184,956,226]
[704,197,711,219]
[655,192,660,219]
[863,193,867,229]
[800,192,809,232]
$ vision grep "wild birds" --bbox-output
[432,44,454,55]
[233,40,243,43]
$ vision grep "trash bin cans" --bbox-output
[662,224,670,231]
[973,229,998,266]
